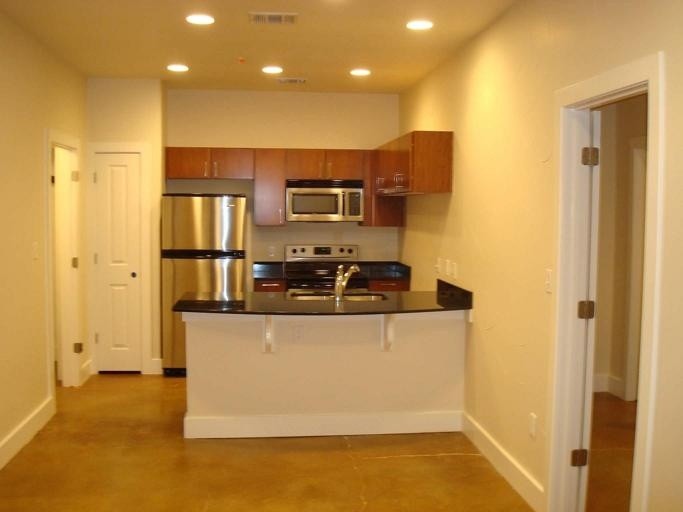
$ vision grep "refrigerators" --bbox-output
[161,194,246,376]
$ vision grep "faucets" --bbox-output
[335,263,360,302]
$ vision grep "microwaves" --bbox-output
[284,179,364,222]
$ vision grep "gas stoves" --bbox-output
[284,269,367,288]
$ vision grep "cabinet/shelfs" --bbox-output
[254,148,286,226]
[364,150,404,226]
[369,280,405,291]
[286,149,365,180]
[254,280,286,292]
[165,147,255,180]
[379,132,454,196]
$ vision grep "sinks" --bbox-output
[342,291,388,302]
[284,287,336,302]
